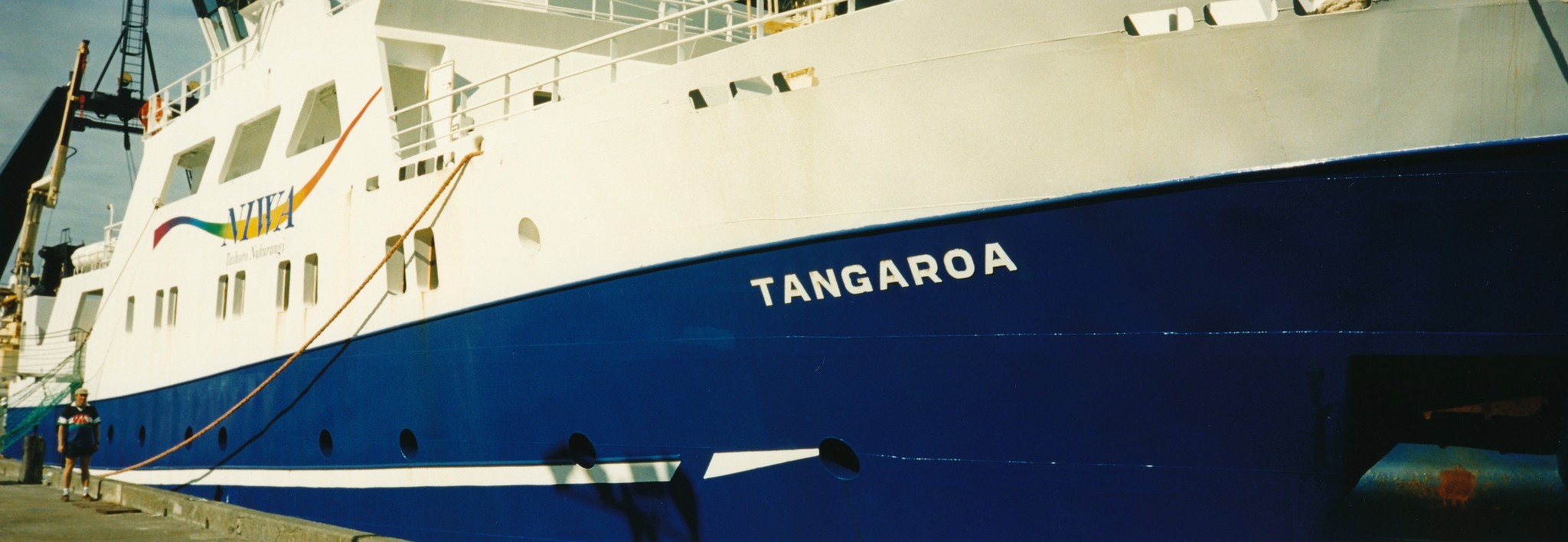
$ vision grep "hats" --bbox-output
[75,388,88,395]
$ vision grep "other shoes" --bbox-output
[82,494,93,501]
[61,495,70,502]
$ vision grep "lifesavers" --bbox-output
[141,98,165,133]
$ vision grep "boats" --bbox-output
[1,0,1568,541]
[69,237,118,268]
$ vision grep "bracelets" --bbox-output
[95,441,99,445]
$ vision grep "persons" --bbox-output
[56,389,101,502]
